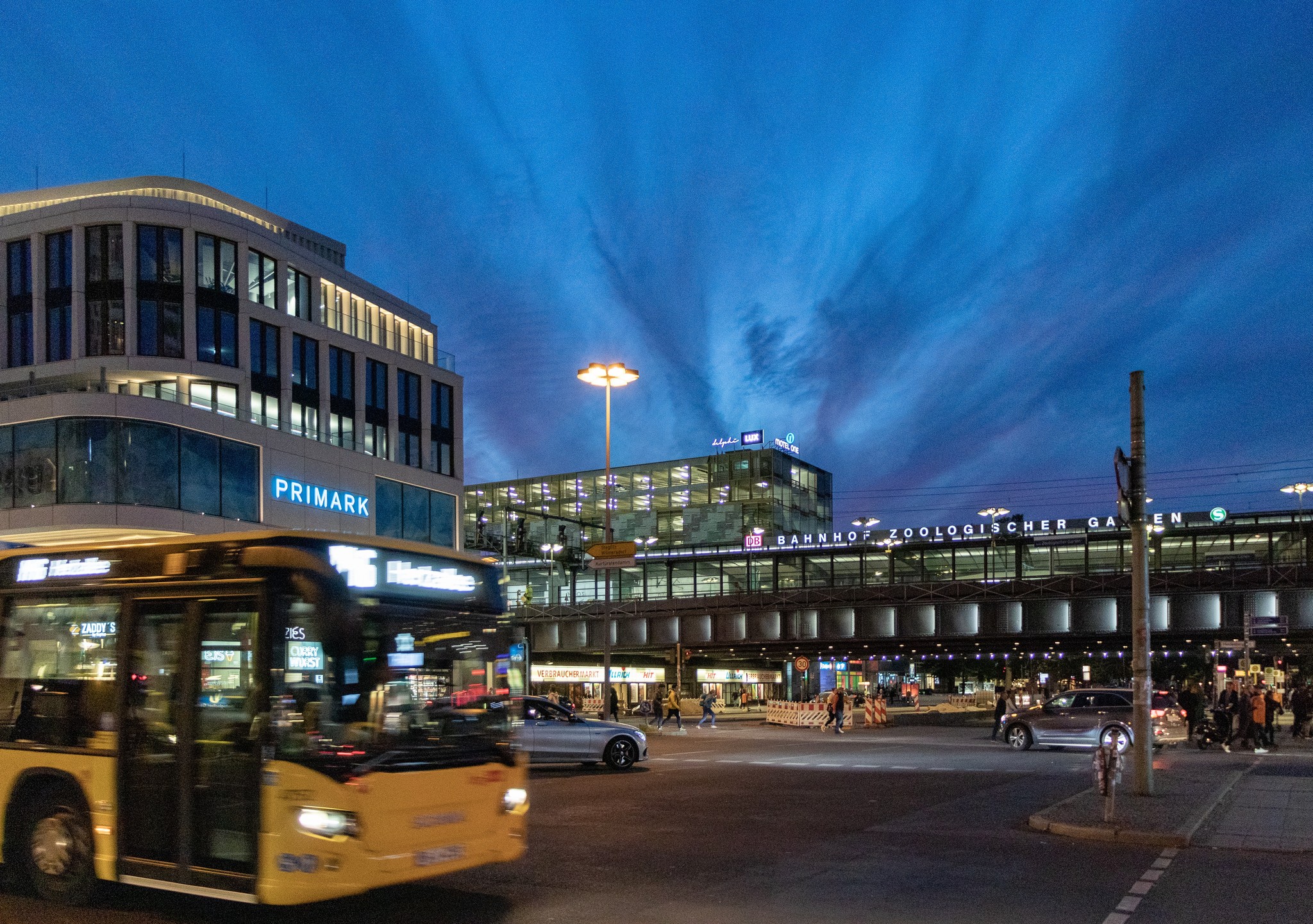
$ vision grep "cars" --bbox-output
[810,692,848,704]
[530,694,576,717]
[995,687,1189,756]
[409,696,649,771]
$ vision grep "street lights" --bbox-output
[578,363,641,721]
[850,517,881,587]
[976,507,1010,582]
[1279,483,1313,588]
[541,544,563,606]
[738,526,765,594]
[634,537,659,600]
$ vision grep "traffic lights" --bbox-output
[685,648,691,660]
[665,648,675,664]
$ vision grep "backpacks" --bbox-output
[700,693,707,699]
[699,701,704,706]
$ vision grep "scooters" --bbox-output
[1193,703,1230,750]
[747,696,761,712]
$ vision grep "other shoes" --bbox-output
[835,730,842,734]
[1254,747,1269,753]
[710,725,717,728]
[679,727,686,731]
[839,728,844,733]
[696,725,701,729]
[821,724,826,732]
[1221,743,1231,753]
[657,726,662,730]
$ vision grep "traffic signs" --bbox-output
[585,541,637,571]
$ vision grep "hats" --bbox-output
[658,683,665,688]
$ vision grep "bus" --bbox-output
[958,681,994,695]
[1,530,527,911]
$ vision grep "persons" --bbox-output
[657,684,686,731]
[738,688,747,709]
[610,687,620,723]
[832,687,844,734]
[926,686,933,695]
[1004,690,1018,714]
[487,688,492,695]
[1288,683,1306,738]
[648,683,666,730]
[585,690,592,699]
[732,688,738,708]
[821,688,844,733]
[877,683,902,706]
[1179,683,1208,742]
[992,693,1008,740]
[696,689,717,729]
[1218,681,1284,753]
[548,684,560,715]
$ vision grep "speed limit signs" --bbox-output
[795,657,809,672]
